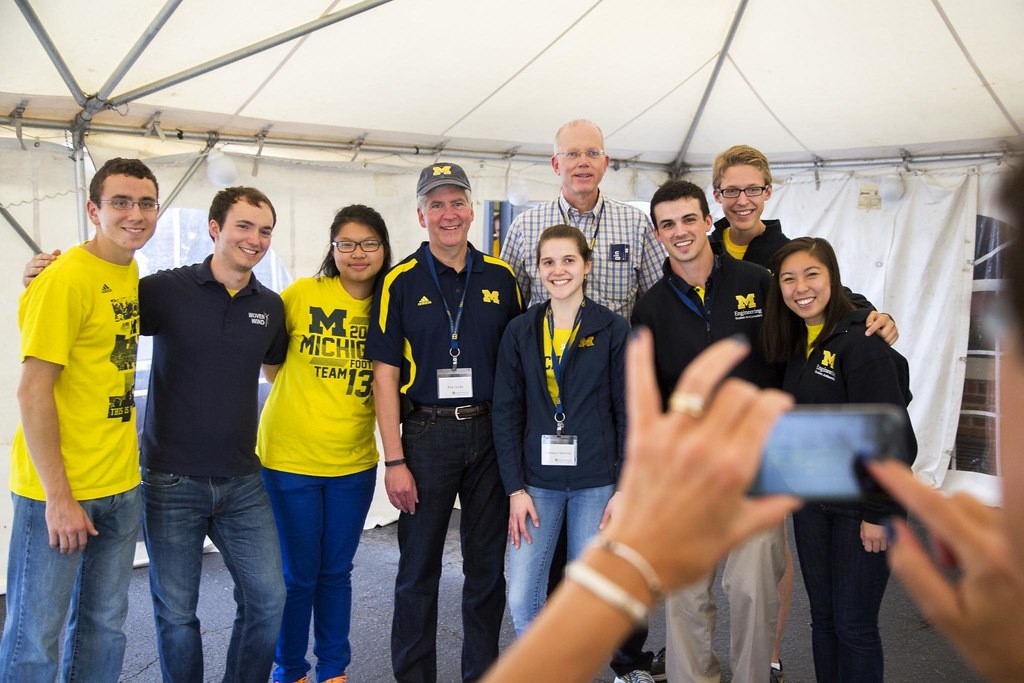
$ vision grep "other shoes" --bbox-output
[650,646,666,681]
[770,659,784,683]
[610,650,654,674]
[614,670,655,683]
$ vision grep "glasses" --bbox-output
[93,198,160,210]
[717,185,769,198]
[556,148,605,160]
[331,240,385,253]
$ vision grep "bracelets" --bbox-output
[565,561,647,624]
[384,458,406,467]
[510,490,525,497]
[584,537,664,603]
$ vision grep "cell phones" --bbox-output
[746,403,909,501]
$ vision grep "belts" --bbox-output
[414,403,489,421]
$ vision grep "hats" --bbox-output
[416,163,471,196]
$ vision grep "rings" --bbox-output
[671,392,705,418]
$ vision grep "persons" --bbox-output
[24,186,289,683]
[0,158,161,683]
[256,121,913,683]
[475,136,1024,683]
